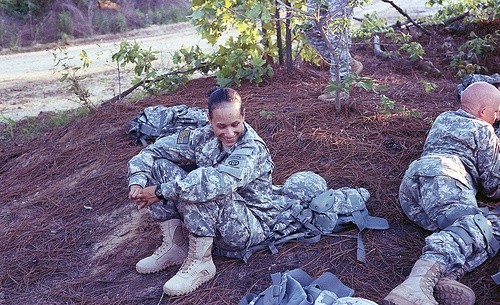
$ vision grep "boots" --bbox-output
[348,53,363,85]
[136,219,189,273]
[383,259,475,305]
[163,236,216,296]
[318,76,349,101]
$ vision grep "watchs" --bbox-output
[152,183,168,209]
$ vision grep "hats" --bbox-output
[282,172,327,201]
[314,289,380,305]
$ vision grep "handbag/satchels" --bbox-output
[314,187,368,234]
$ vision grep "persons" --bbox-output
[380,81,500,305]
[125,88,275,297]
[302,0,363,102]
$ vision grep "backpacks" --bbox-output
[126,105,209,146]
[213,193,316,262]
[456,73,500,100]
